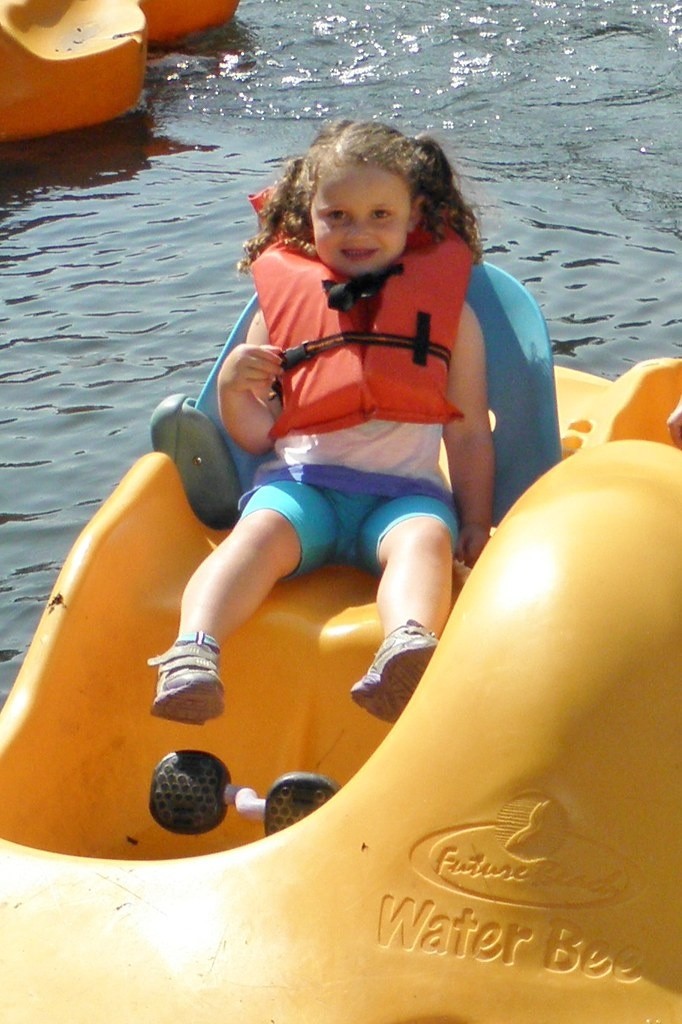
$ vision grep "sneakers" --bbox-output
[148,631,225,725]
[351,619,440,723]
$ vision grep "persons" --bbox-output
[149,119,494,724]
